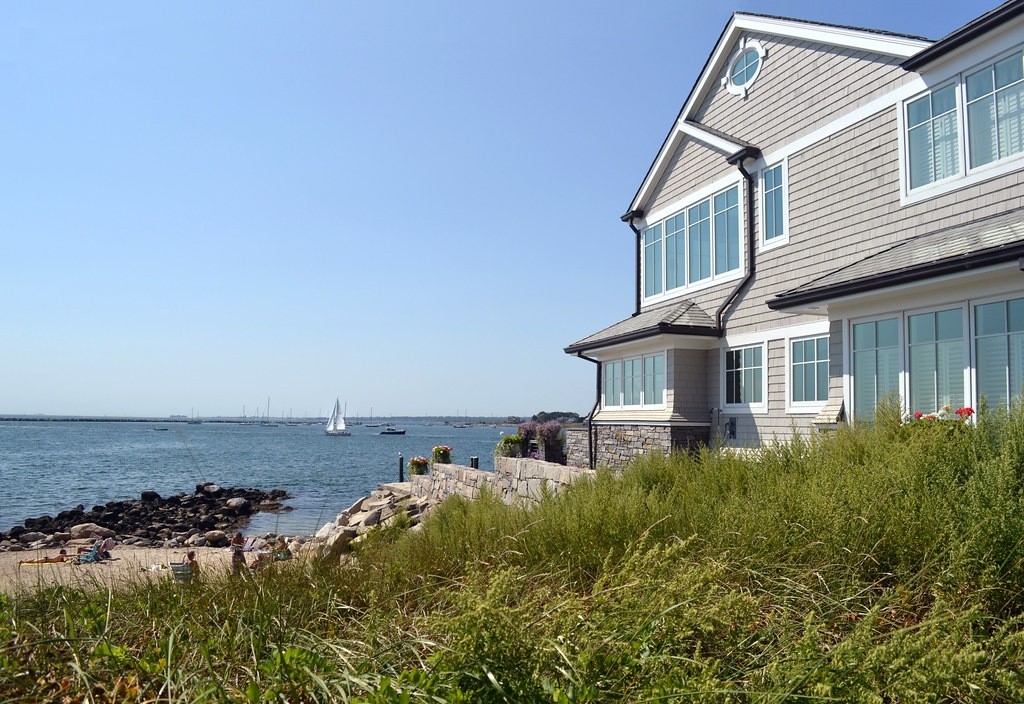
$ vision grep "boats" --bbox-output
[378,422,407,435]
[421,408,497,428]
[154,428,168,431]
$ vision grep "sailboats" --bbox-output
[187,408,201,424]
[239,395,396,427]
[324,397,352,436]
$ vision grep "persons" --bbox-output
[231,533,246,564]
[20,550,80,563]
[250,534,304,569]
[77,535,109,554]
[186,551,201,574]
